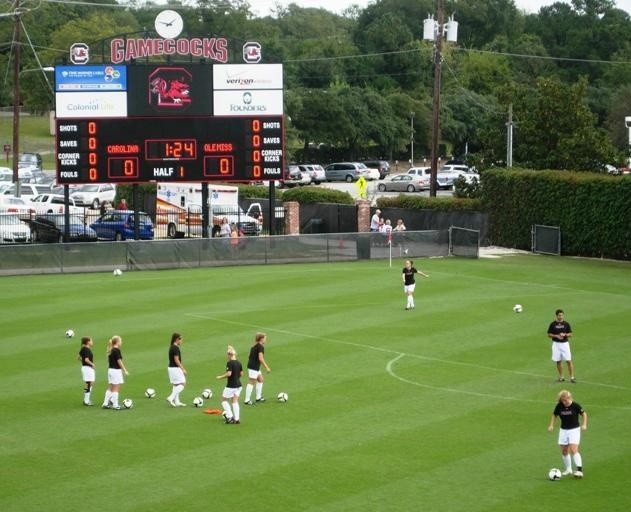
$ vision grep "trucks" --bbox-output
[157,184,263,236]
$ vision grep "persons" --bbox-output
[401,261,429,310]
[216,346,244,425]
[212,218,245,253]
[76,338,97,407]
[549,390,588,478]
[371,209,407,248]
[166,333,188,407]
[245,332,271,406]
[100,198,127,213]
[102,336,129,410]
[548,310,577,384]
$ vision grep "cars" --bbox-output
[598,161,630,175]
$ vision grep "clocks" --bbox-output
[155,9,184,39]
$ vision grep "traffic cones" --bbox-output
[336,236,346,249]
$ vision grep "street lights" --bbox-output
[421,13,459,196]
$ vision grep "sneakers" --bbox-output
[559,377,564,382]
[84,397,265,423]
[571,379,576,383]
[562,470,572,476]
[574,471,583,478]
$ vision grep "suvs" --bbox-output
[230,161,505,192]
[0,154,155,243]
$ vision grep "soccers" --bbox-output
[193,397,203,407]
[278,392,288,402]
[202,389,212,398]
[114,269,122,277]
[66,330,74,338]
[145,388,156,398]
[123,399,133,409]
[549,468,561,481]
[514,304,523,313]
